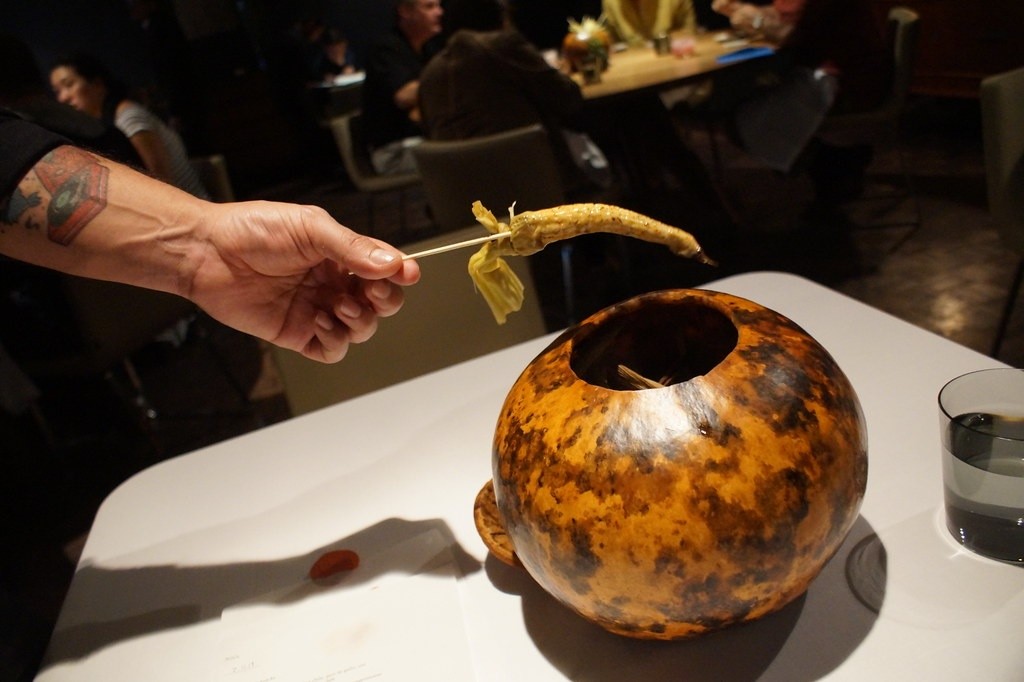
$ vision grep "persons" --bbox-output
[0,35,148,177]
[420,0,578,143]
[48,49,284,403]
[598,0,864,218]
[364,0,444,145]
[0,110,420,364]
[305,28,419,196]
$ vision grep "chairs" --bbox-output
[975,62,1023,357]
[64,267,255,445]
[189,157,235,202]
[330,107,442,243]
[265,216,554,413]
[412,122,616,325]
[806,7,925,237]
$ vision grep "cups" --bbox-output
[938,367,1024,566]
[843,505,1023,634]
[653,35,672,56]
[577,54,604,86]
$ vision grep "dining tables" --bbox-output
[29,270,1023,682]
[542,25,780,182]
